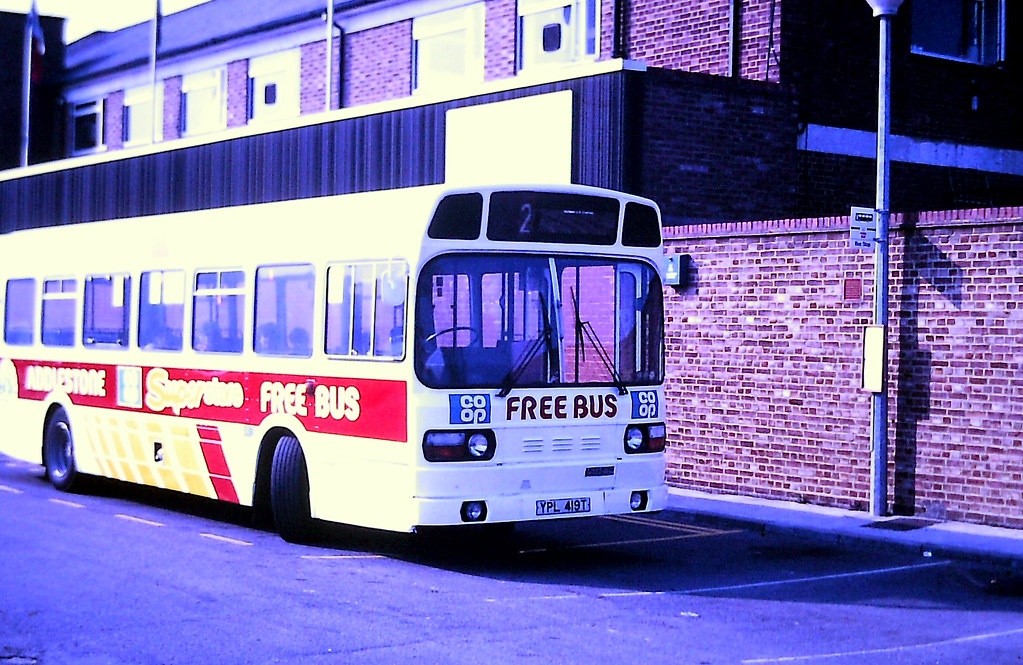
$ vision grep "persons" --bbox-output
[141,322,310,356]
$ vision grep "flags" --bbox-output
[31,10,45,55]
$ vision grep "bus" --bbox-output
[0,181,670,545]
